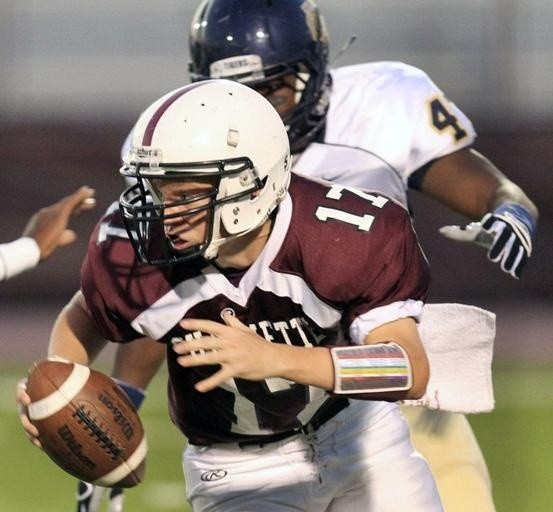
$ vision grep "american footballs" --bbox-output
[25,360,148,487]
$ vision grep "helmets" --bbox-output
[119,77,287,237]
[188,0,333,153]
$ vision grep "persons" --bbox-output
[15,77,443,511]
[0,184,98,281]
[185,1,539,512]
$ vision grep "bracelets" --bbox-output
[325,342,411,403]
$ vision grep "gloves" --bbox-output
[436,202,538,281]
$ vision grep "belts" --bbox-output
[187,398,351,448]
